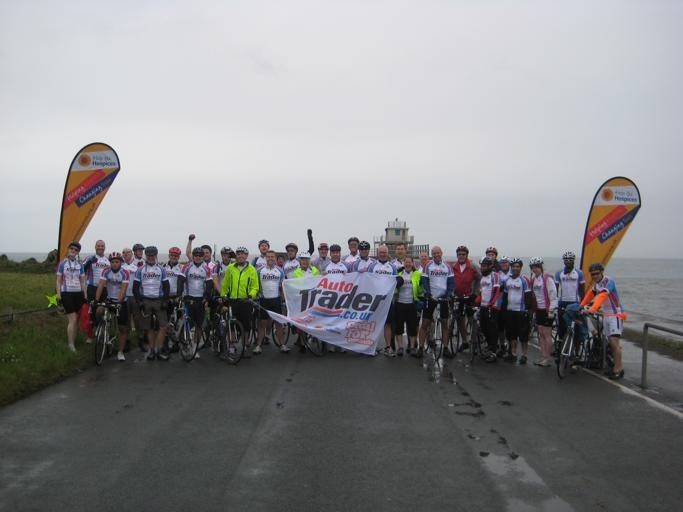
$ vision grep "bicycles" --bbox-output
[91,297,118,365]
[554,307,601,380]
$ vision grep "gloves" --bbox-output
[189,234,195,240]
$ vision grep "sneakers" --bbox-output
[117,351,126,361]
[145,336,553,367]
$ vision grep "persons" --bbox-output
[578,263,624,377]
[57,229,587,369]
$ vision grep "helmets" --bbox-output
[68,237,604,271]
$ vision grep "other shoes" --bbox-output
[70,344,77,351]
[85,338,93,343]
